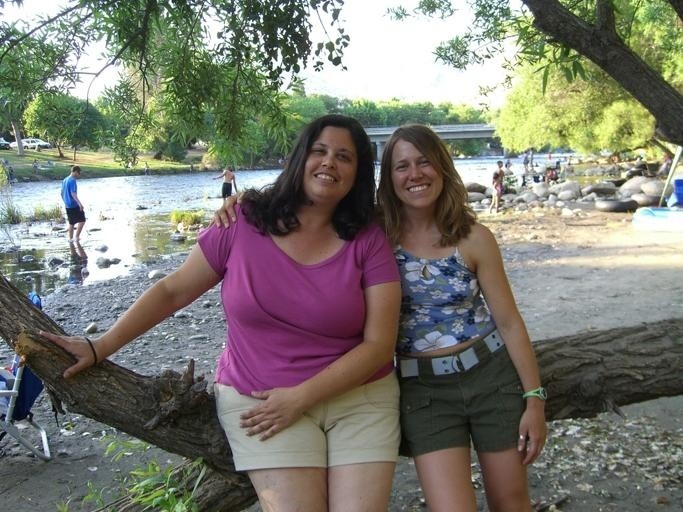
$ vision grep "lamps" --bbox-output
[0,292,51,464]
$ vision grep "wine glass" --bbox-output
[519,435,526,441]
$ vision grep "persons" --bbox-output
[658,150,673,176]
[212,164,241,203]
[38,114,404,511]
[486,146,573,215]
[59,162,87,238]
[212,124,552,511]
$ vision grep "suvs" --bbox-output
[675,179,683,203]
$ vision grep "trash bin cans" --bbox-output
[523,384,549,403]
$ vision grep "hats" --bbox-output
[0,137,10,150]
[9,138,52,150]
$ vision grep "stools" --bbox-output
[397,328,506,384]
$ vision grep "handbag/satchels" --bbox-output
[83,335,101,367]
